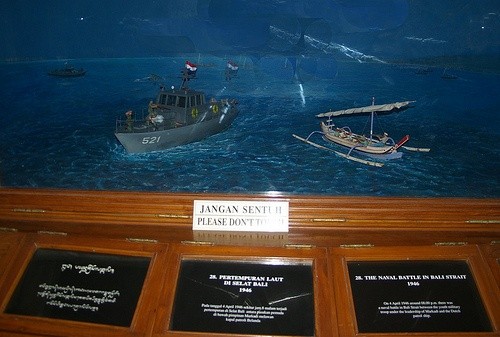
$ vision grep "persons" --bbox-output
[154,112,164,127]
[377,132,388,143]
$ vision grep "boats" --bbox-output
[114,61,238,153]
[293,98,431,168]
[47,62,88,77]
